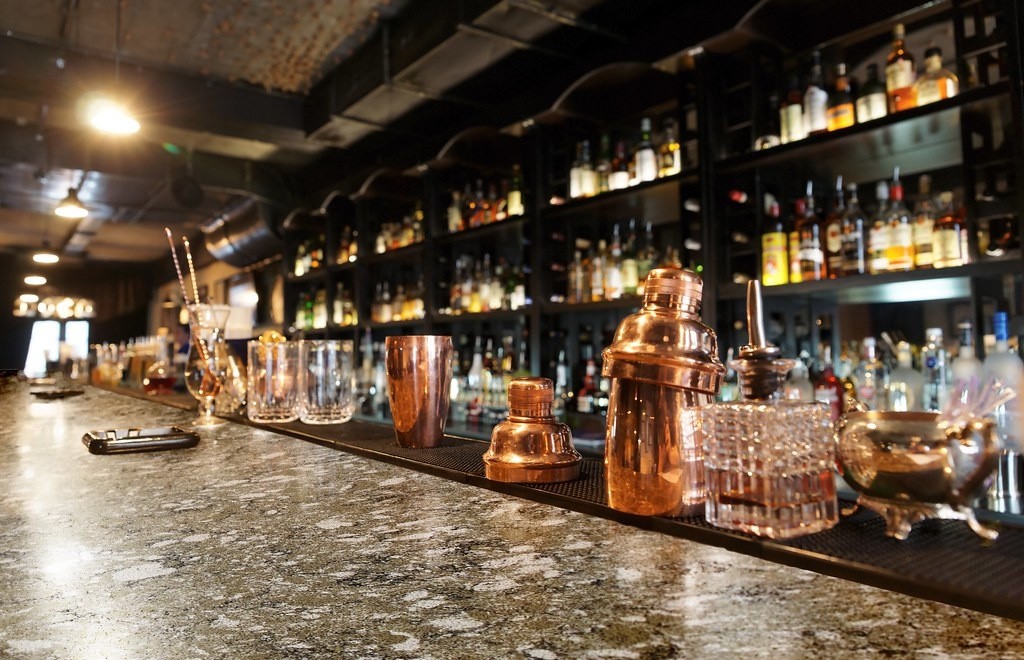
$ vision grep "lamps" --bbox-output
[53,172,92,217]
[31,239,60,264]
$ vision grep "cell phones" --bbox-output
[30,390,85,400]
[82,425,201,455]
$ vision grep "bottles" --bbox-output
[296,278,425,330]
[963,17,1007,90]
[971,119,1010,200]
[726,313,1024,443]
[553,324,617,408]
[754,21,958,150]
[566,116,682,201]
[977,214,1014,252]
[763,165,965,288]
[444,162,524,233]
[548,218,657,300]
[599,267,724,519]
[294,197,426,276]
[435,253,523,314]
[355,332,529,405]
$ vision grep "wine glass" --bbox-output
[182,304,231,429]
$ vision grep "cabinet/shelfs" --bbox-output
[280,0,1024,379]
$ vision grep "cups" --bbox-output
[298,339,351,423]
[385,335,454,449]
[247,341,299,423]
[700,401,840,539]
